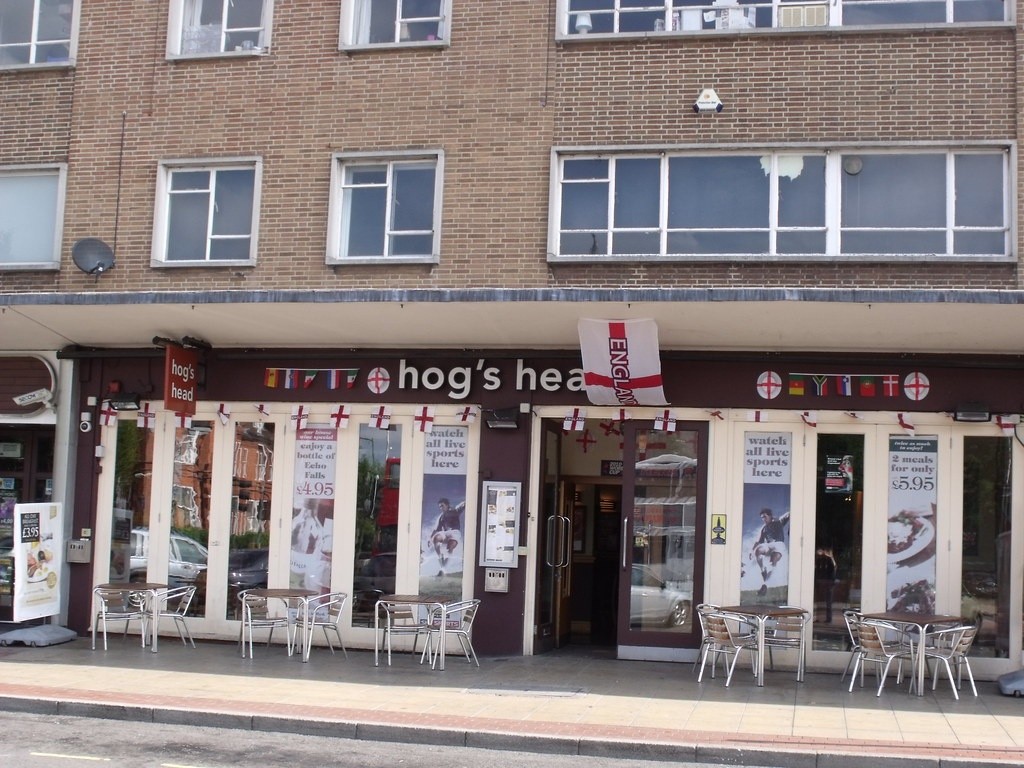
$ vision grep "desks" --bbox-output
[91,581,170,653]
[857,611,965,697]
[713,604,809,686]
[375,593,453,671]
[241,589,320,663]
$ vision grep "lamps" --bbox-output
[108,393,141,411]
[953,410,991,422]
[575,13,592,34]
[484,410,519,429]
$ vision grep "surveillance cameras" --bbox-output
[12,388,52,406]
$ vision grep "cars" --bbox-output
[630,564,692,627]
[128,529,208,609]
[961,571,997,641]
[352,551,396,619]
[195,548,268,609]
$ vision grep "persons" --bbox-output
[749,509,790,596]
[838,455,853,492]
[815,548,837,623]
[428,498,466,577]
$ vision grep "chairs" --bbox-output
[352,589,384,628]
[694,602,980,701]
[91,585,481,671]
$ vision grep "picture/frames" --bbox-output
[573,504,586,554]
